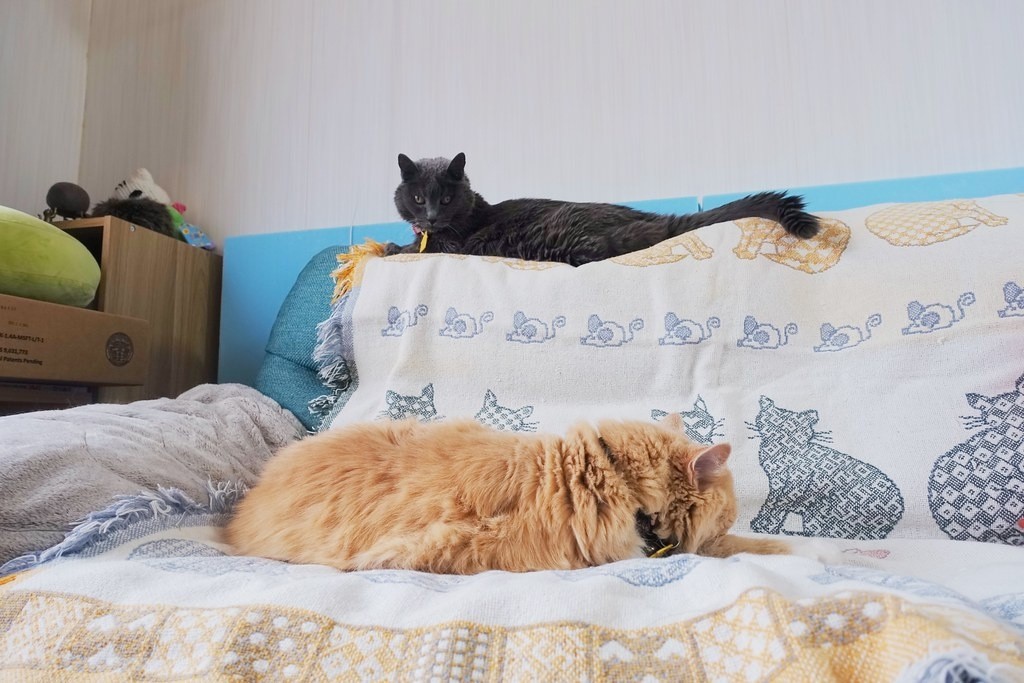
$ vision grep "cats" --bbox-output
[382,151,822,260]
[217,413,792,566]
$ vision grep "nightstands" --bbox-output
[39,215,224,411]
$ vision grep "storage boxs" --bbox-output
[1,293,153,387]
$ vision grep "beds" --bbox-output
[1,162,1023,683]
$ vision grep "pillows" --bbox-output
[0,205,101,307]
[251,238,365,430]
